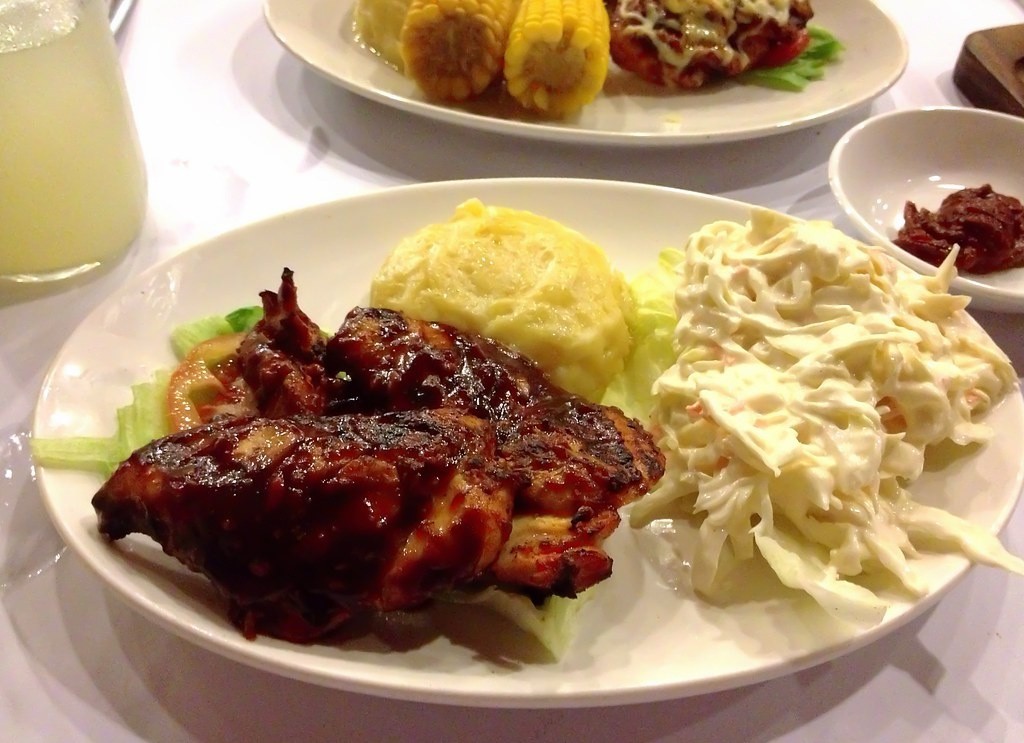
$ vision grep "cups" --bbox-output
[0,0,150,285]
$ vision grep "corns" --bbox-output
[399,0,612,114]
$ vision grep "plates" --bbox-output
[260,2,911,146]
[30,176,1023,708]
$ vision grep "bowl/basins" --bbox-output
[829,107,1023,318]
